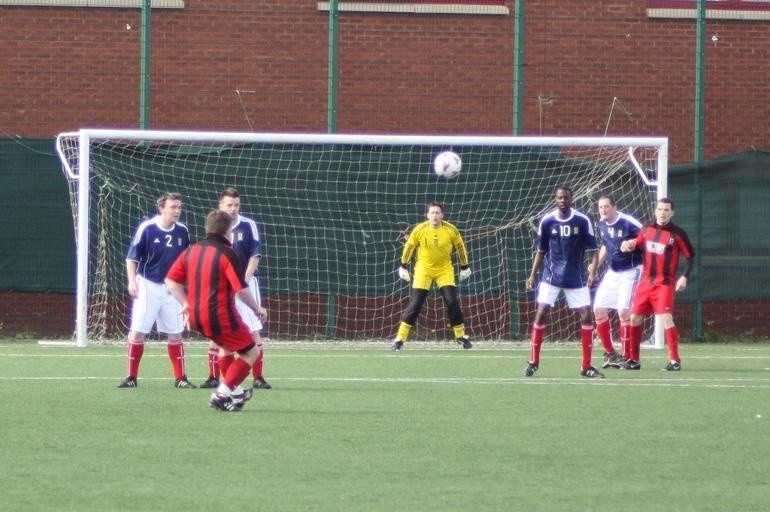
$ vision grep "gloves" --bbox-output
[398,266,412,282]
[459,268,471,282]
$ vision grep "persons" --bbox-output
[117,192,197,388]
[199,188,272,389]
[164,210,268,411]
[620,199,695,371]
[525,186,605,378]
[588,195,645,368]
[392,202,472,350]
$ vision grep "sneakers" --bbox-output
[209,387,252,411]
[663,360,681,371]
[174,378,195,389]
[117,375,136,388]
[253,376,270,389]
[523,362,539,376]
[393,340,403,351]
[580,366,603,378]
[455,336,472,348]
[601,352,641,370]
[200,376,219,388]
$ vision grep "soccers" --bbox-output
[434,151,462,179]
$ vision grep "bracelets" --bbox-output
[401,264,407,269]
[460,266,469,270]
